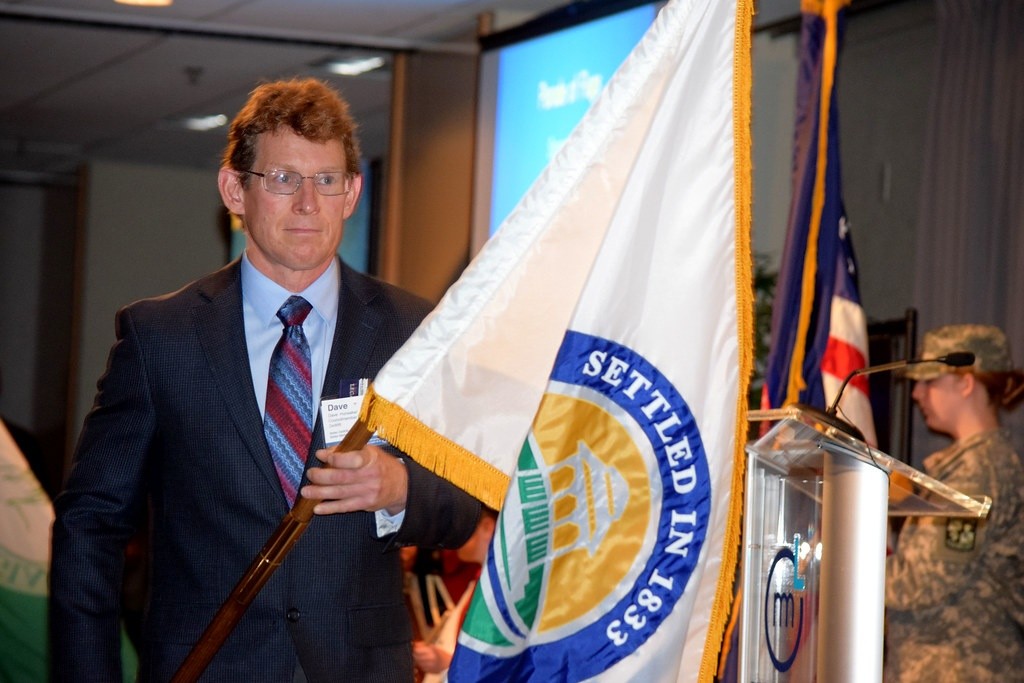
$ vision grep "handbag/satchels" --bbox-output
[423,573,476,683]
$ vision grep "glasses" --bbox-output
[236,166,354,198]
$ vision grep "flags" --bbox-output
[754,2,881,462]
[0,415,145,683]
[348,0,760,682]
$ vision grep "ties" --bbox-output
[261,295,313,510]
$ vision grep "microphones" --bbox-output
[788,352,980,441]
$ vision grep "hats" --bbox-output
[902,323,1014,383]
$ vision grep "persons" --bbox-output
[871,317,1024,680]
[45,73,485,683]
